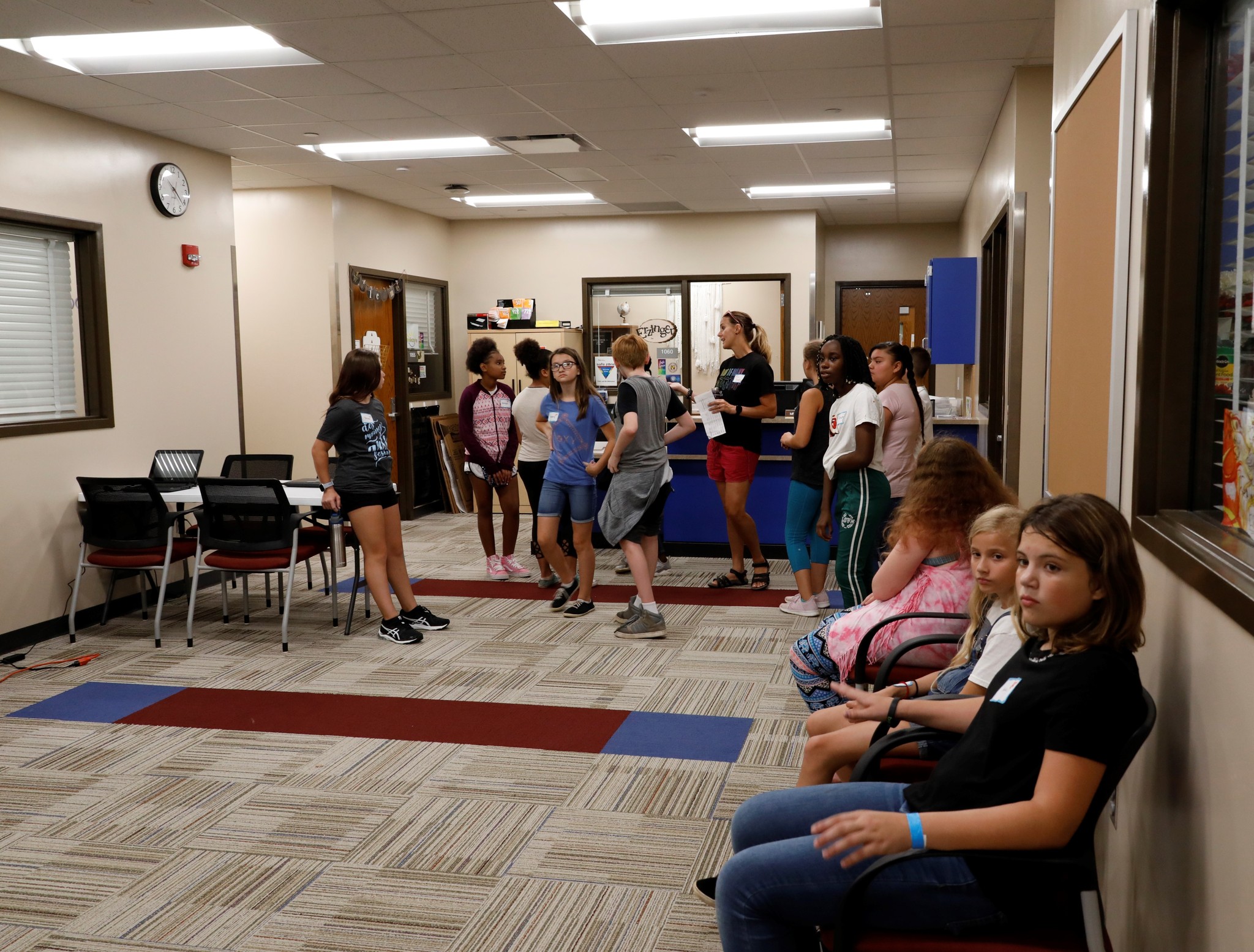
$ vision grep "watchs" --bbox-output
[735,405,743,415]
[319,481,335,492]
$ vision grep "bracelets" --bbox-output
[905,811,927,849]
[910,678,919,698]
[685,388,693,399]
[899,681,909,699]
[887,697,902,728]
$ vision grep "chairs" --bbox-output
[818,686,1162,952]
[848,610,973,682]
[218,454,296,627]
[851,630,963,768]
[311,457,377,619]
[185,474,340,655]
[65,474,215,649]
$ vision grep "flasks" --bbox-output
[329,512,346,568]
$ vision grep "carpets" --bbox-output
[314,571,848,614]
[5,679,758,765]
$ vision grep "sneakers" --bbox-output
[378,614,424,644]
[399,604,450,631]
[615,594,642,624]
[653,555,673,577]
[779,595,820,617]
[486,554,509,579]
[500,554,531,577]
[615,554,631,573]
[563,597,595,617]
[784,587,830,608]
[549,577,580,612]
[614,609,668,639]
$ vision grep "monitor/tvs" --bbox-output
[773,381,803,416]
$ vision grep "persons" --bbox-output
[818,334,890,610]
[664,309,778,591]
[613,356,675,577]
[511,338,598,587]
[777,339,839,619]
[312,347,450,645]
[788,435,1020,781]
[713,494,1145,952]
[608,333,697,640]
[867,341,923,564]
[536,347,616,617]
[905,345,933,461]
[693,503,1028,908]
[459,337,532,580]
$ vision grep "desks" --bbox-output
[76,478,403,638]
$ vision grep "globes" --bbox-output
[617,301,631,317]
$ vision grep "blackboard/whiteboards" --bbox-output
[593,330,613,355]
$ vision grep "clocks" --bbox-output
[148,161,191,219]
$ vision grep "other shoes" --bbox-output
[538,573,562,588]
[692,874,718,908]
[578,578,598,588]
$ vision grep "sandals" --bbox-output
[751,557,770,590]
[707,568,748,589]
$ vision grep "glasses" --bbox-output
[722,310,740,324]
[550,361,578,372]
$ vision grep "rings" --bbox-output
[718,405,719,409]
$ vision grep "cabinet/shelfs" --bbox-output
[465,328,584,516]
[921,256,978,367]
